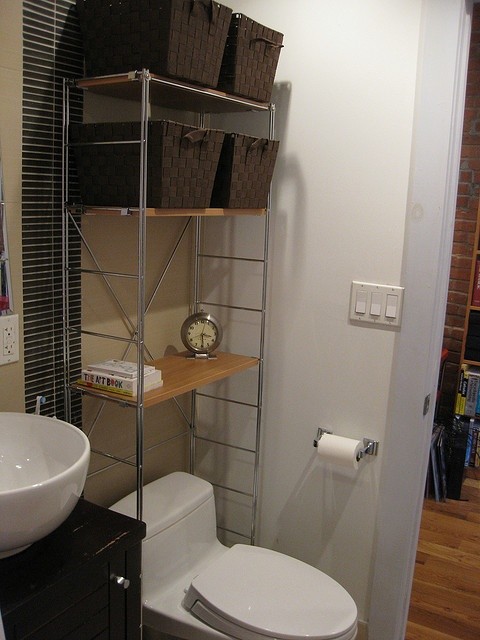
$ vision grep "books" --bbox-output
[454,363,480,417]
[75,359,164,397]
[463,419,480,469]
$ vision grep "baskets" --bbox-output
[69,119,226,209]
[209,132,280,212]
[216,13,285,103]
[77,0,233,81]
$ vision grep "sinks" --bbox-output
[0,412,92,563]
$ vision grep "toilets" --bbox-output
[108,471,358,640]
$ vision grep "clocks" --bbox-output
[180,311,224,354]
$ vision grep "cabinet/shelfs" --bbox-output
[452,200,480,483]
[0,499,143,639]
[61,71,277,567]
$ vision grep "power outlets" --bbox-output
[1,316,21,364]
